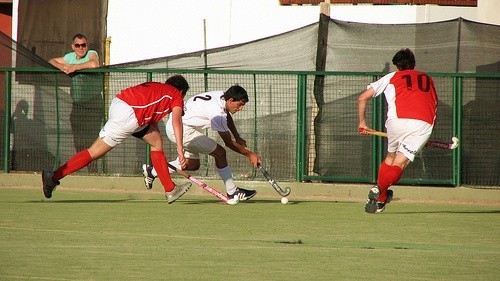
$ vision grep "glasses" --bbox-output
[72,43,87,48]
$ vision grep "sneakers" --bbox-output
[227,187,256,201]
[142,164,155,190]
[42,170,60,198]
[165,182,192,204]
[376,190,393,212]
[365,185,382,213]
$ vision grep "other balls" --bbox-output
[280,197,289,205]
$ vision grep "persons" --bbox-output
[47,34,102,174]
[357,47,439,213]
[42,74,192,204]
[142,83,262,202]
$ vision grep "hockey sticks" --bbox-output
[360,128,458,149]
[257,162,291,196]
[166,162,239,205]
